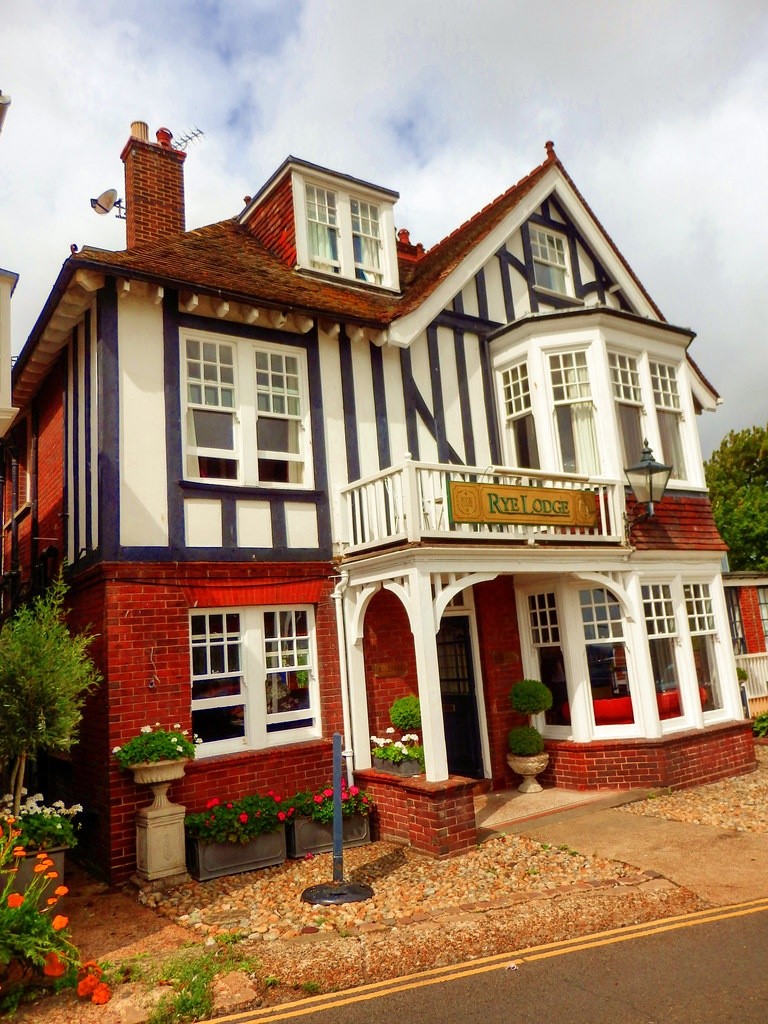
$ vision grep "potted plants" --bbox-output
[752,710,768,766]
[737,666,748,691]
[506,679,553,794]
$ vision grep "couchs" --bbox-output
[559,685,708,726]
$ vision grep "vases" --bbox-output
[1,841,71,922]
[125,755,192,786]
[184,808,373,882]
[372,755,422,778]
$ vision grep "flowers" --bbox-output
[0,787,83,852]
[184,775,375,844]
[111,722,204,774]
[0,815,113,1018]
[370,726,425,762]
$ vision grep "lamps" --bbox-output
[623,437,675,538]
